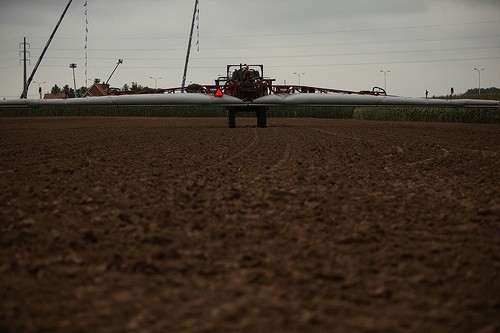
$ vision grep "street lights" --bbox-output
[474,66,486,95]
[33,81,46,100]
[380,70,391,96]
[150,76,162,91]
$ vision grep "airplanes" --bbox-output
[1,63,500,127]
